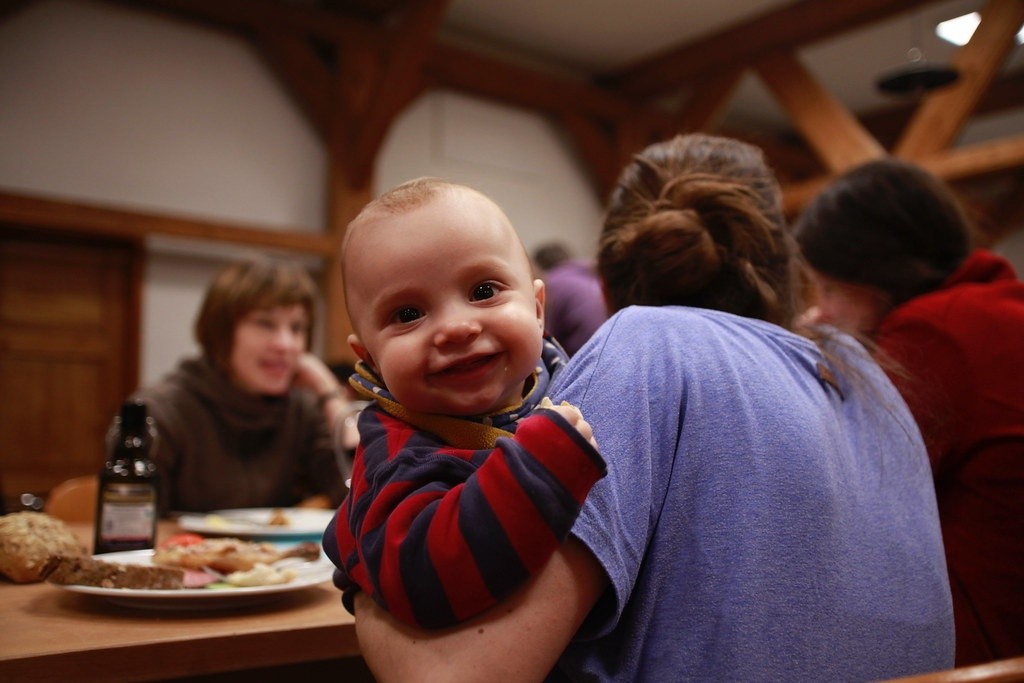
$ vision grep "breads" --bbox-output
[0,509,186,591]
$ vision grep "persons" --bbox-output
[793,159,1023,669]
[97,255,360,511]
[324,176,611,683]
[330,131,956,683]
[527,239,612,365]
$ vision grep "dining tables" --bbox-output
[0,526,361,683]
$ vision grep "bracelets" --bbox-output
[316,383,350,414]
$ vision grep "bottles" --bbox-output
[91,397,159,556]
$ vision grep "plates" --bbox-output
[48,549,337,609]
[179,507,339,540]
